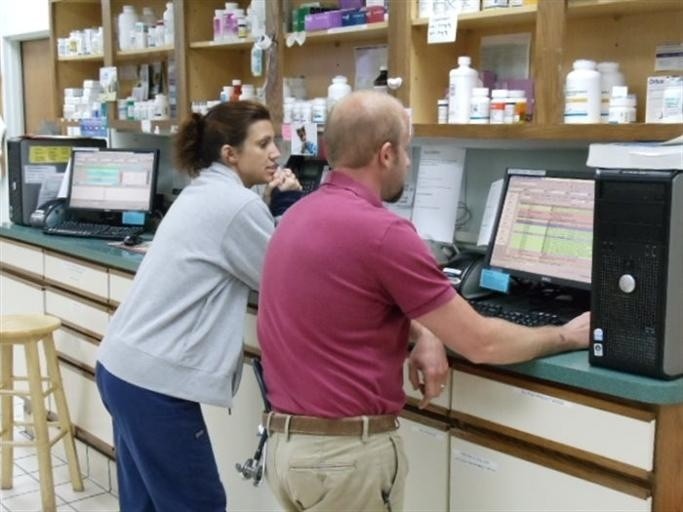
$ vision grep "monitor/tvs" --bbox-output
[42,147,159,222]
[486,167,596,291]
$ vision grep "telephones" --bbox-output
[283,156,328,199]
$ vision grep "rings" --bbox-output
[440,384,445,389]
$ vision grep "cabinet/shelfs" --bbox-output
[49,0,683,142]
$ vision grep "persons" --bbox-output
[255,90,593,512]
[96,100,303,512]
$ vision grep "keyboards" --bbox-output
[43,218,145,240]
[460,291,567,340]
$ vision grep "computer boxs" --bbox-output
[7,137,106,227]
[587,166,683,382]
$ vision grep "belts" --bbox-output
[263,412,396,434]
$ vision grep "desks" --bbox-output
[3,234,683,512]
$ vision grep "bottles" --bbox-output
[57,1,683,125]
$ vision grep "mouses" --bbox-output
[123,234,141,247]
